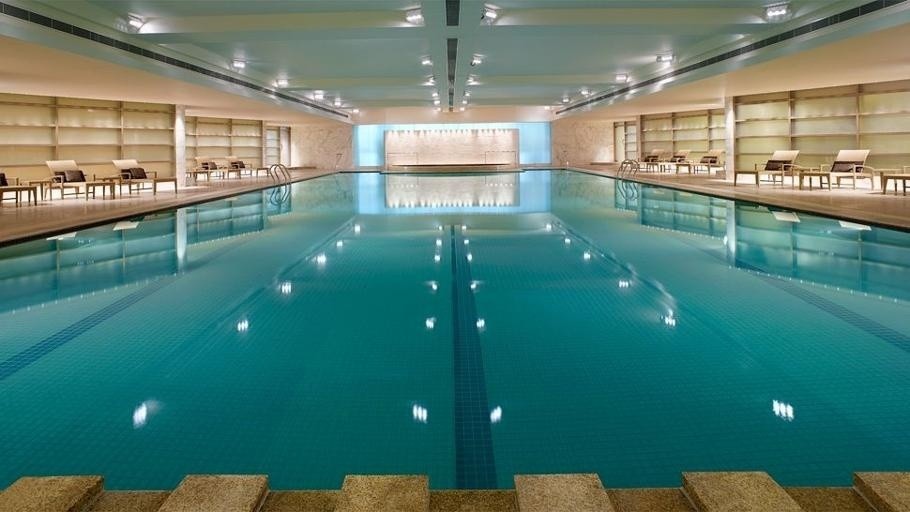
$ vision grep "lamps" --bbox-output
[545,52,674,115]
[660,308,678,328]
[411,221,503,429]
[126,12,155,34]
[402,3,499,116]
[765,4,793,21]
[772,398,793,420]
[543,223,632,291]
[280,223,361,295]
[132,401,152,428]
[228,61,362,113]
[234,319,249,332]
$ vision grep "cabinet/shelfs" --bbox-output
[0,93,177,186]
[637,108,726,163]
[0,182,292,314]
[265,127,281,167]
[625,121,637,161]
[614,179,910,304]
[733,79,910,174]
[185,115,265,174]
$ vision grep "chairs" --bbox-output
[186,156,269,182]
[638,149,910,196]
[0,159,178,208]
